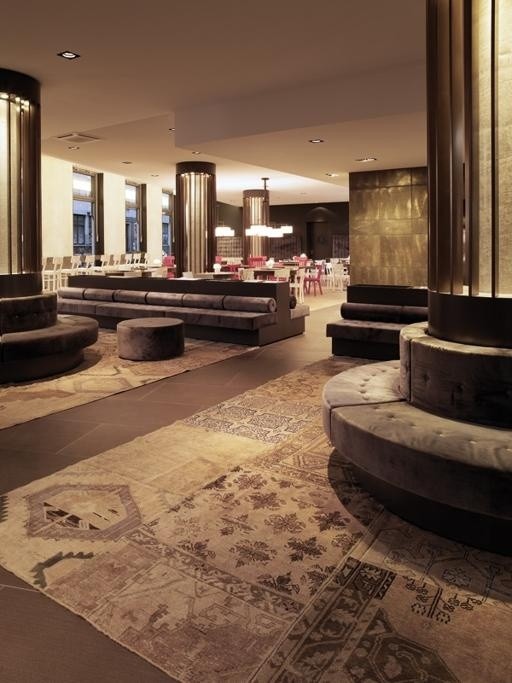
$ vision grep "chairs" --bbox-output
[42,253,350,304]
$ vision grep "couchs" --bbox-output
[0,275,512,560]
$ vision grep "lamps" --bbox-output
[215,178,293,238]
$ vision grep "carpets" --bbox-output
[0,327,512,683]
